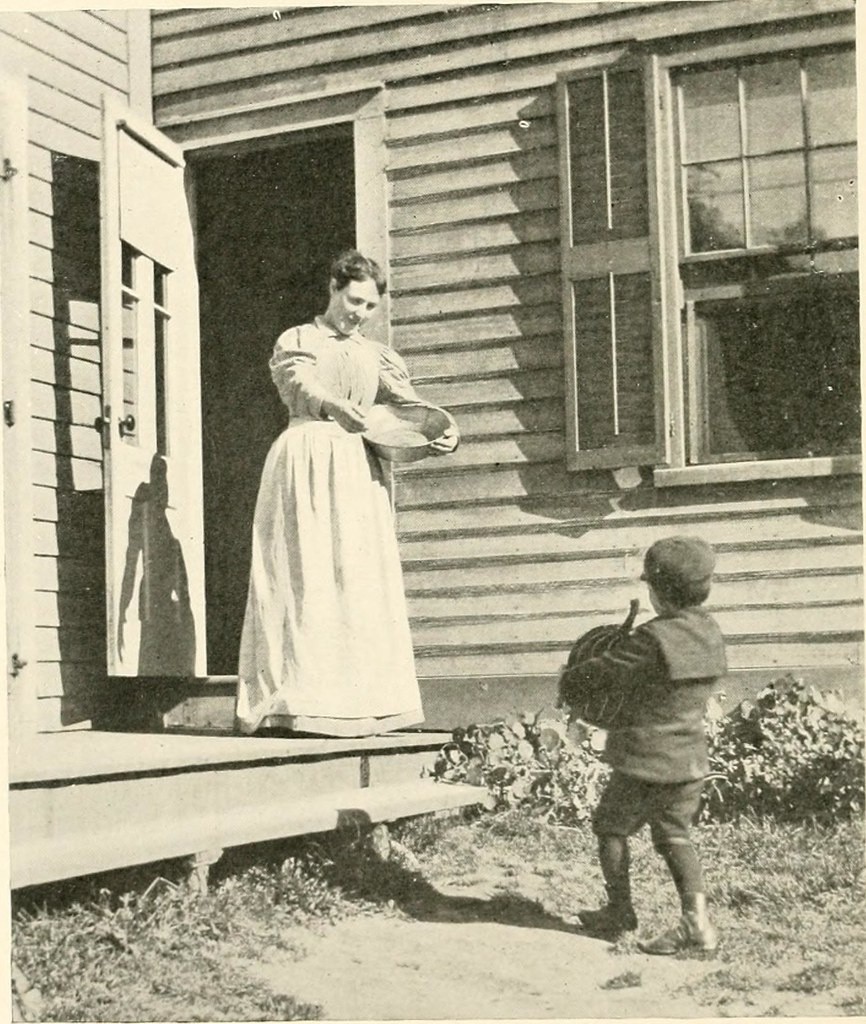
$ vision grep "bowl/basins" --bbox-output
[360,405,450,461]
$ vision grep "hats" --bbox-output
[639,537,714,583]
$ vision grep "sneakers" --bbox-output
[579,902,638,933]
[639,917,717,956]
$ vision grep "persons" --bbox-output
[556,534,729,954]
[234,249,460,738]
